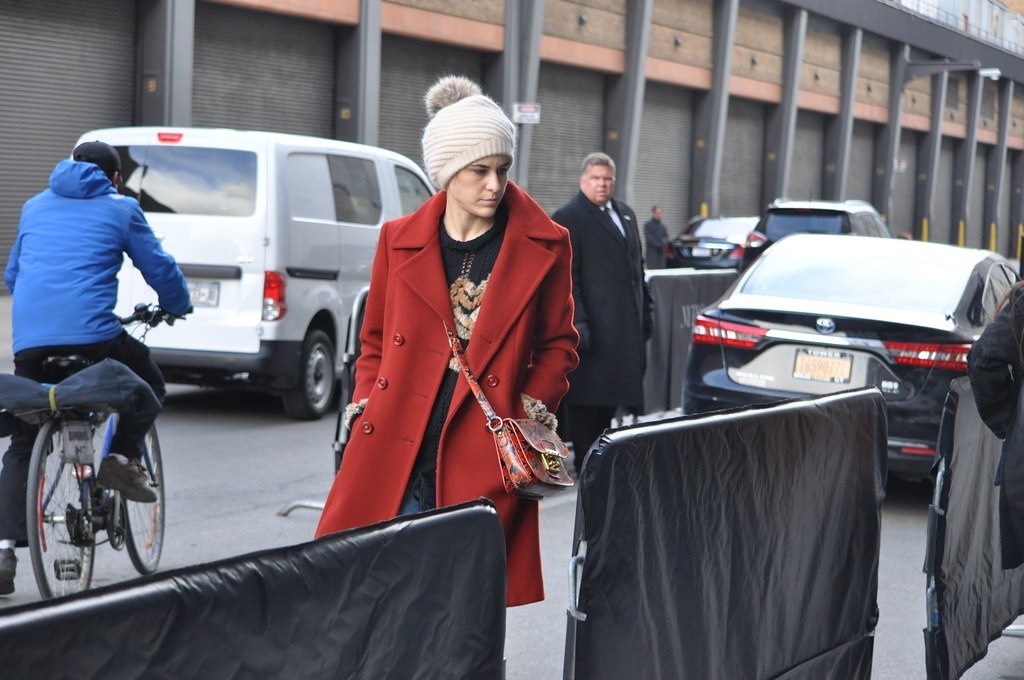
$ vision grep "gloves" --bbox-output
[643,314,653,341]
[573,321,591,352]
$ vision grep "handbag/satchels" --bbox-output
[493,417,574,501]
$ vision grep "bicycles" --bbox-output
[26,304,194,603]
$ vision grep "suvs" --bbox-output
[737,198,909,275]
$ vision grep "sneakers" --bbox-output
[0,548,17,594]
[95,455,158,502]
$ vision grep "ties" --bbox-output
[603,208,625,241]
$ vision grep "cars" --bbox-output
[681,231,1024,483]
[665,218,761,269]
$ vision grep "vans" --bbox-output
[67,125,440,422]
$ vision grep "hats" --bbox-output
[421,74,518,191]
[72,139,121,181]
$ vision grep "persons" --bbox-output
[308,76,580,608]
[0,140,193,595]
[644,206,669,270]
[553,152,656,479]
[965,280,1024,571]
